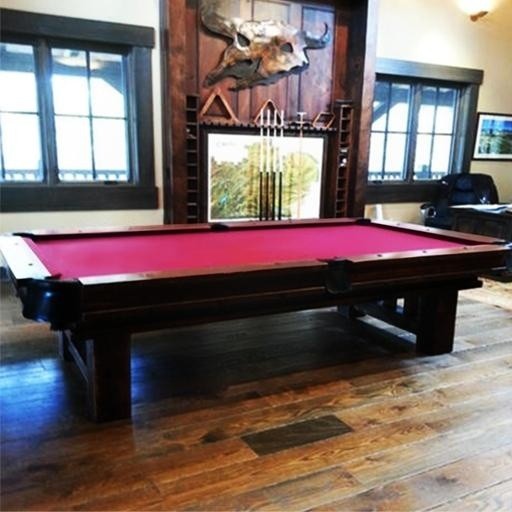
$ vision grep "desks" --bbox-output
[1,217,512,424]
[450,202,511,236]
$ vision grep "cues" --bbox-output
[260,107,285,220]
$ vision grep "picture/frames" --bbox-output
[471,112,512,163]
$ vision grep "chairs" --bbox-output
[419,173,499,227]
